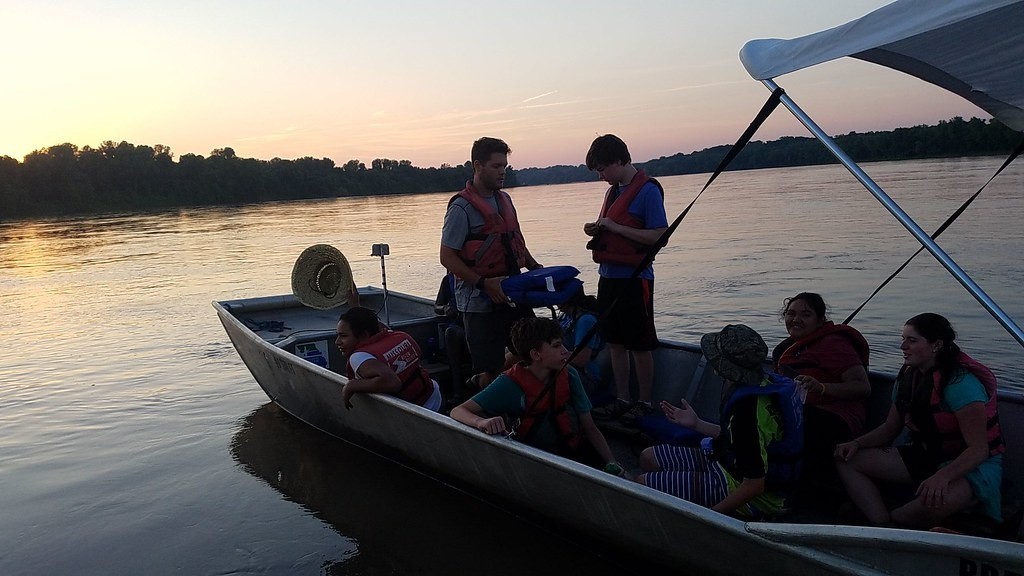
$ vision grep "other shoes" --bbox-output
[603,398,659,419]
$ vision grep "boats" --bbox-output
[209,1,1024,576]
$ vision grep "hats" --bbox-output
[700,324,768,380]
[291,244,354,310]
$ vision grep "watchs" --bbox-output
[474,276,485,290]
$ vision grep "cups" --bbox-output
[424,337,438,364]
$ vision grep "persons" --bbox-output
[584,134,668,427]
[450,317,618,466]
[832,312,1005,527]
[772,292,871,457]
[335,307,446,414]
[433,136,543,396]
[638,323,803,515]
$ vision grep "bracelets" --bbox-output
[819,383,825,395]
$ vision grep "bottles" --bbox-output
[605,460,620,475]
[740,502,751,513]
